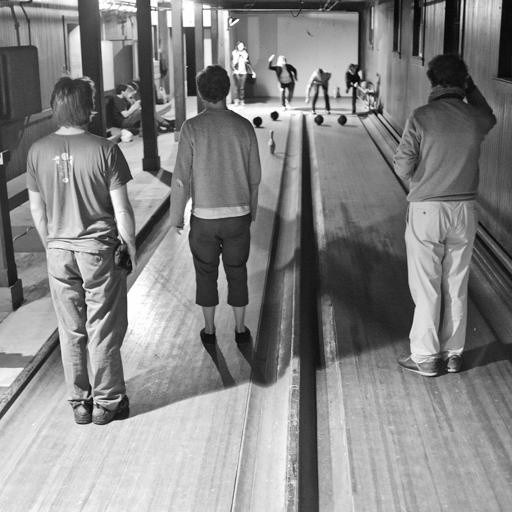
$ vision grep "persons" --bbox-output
[268,55,297,107]
[231,40,256,105]
[26,77,137,425]
[110,81,175,133]
[306,68,331,114]
[345,64,361,114]
[169,64,262,344]
[391,56,497,376]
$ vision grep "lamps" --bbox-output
[227,16,240,31]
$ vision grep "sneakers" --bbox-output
[200,327,216,346]
[396,352,439,376]
[235,324,250,345]
[440,355,463,372]
[73,393,128,424]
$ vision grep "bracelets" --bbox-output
[306,97,311,98]
